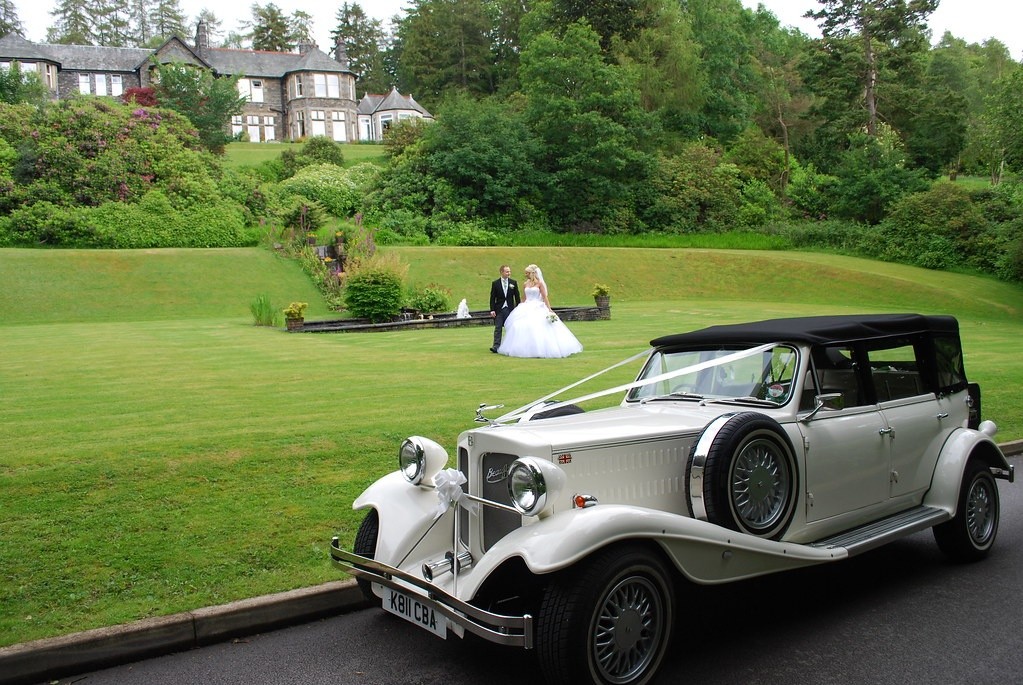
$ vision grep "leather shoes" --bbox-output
[490,346,497,353]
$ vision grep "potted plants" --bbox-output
[591,284,611,307]
[282,302,308,330]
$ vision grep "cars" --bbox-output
[328,307,1016,685]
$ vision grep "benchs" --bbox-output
[805,368,920,402]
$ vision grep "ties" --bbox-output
[503,280,507,290]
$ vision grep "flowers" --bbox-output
[306,232,318,238]
[335,231,344,238]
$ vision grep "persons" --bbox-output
[489,264,521,353]
[498,264,583,359]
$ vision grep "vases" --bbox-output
[336,237,344,243]
[308,237,315,244]
